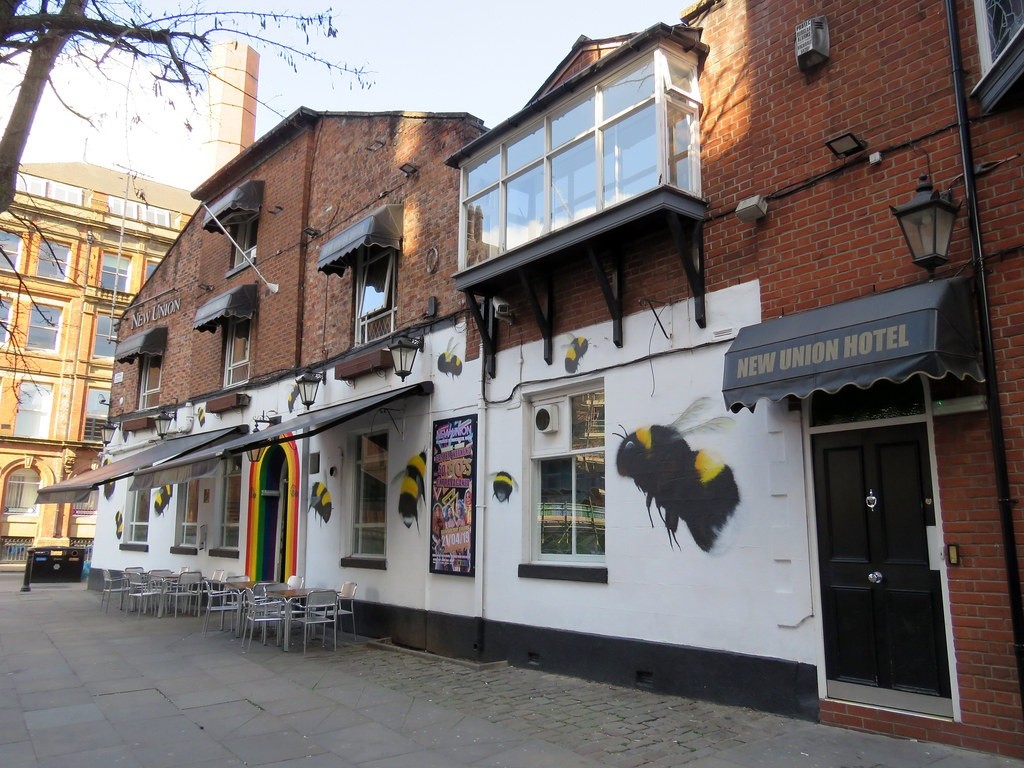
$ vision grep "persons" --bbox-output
[431,489,473,571]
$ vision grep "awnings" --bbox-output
[127,380,435,492]
[113,326,169,365]
[34,424,250,506]
[722,277,987,413]
[317,203,403,278]
[192,282,258,335]
[202,179,266,235]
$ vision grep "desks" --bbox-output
[265,588,341,652]
[142,573,207,619]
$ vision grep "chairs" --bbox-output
[100,567,358,656]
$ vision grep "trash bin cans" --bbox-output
[30,546,85,583]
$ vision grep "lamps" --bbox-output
[246,410,297,463]
[154,408,176,440]
[386,332,424,383]
[198,284,213,291]
[100,419,121,447]
[825,132,865,160]
[303,227,321,238]
[735,195,768,224]
[399,162,420,176]
[295,364,326,410]
[888,172,962,280]
